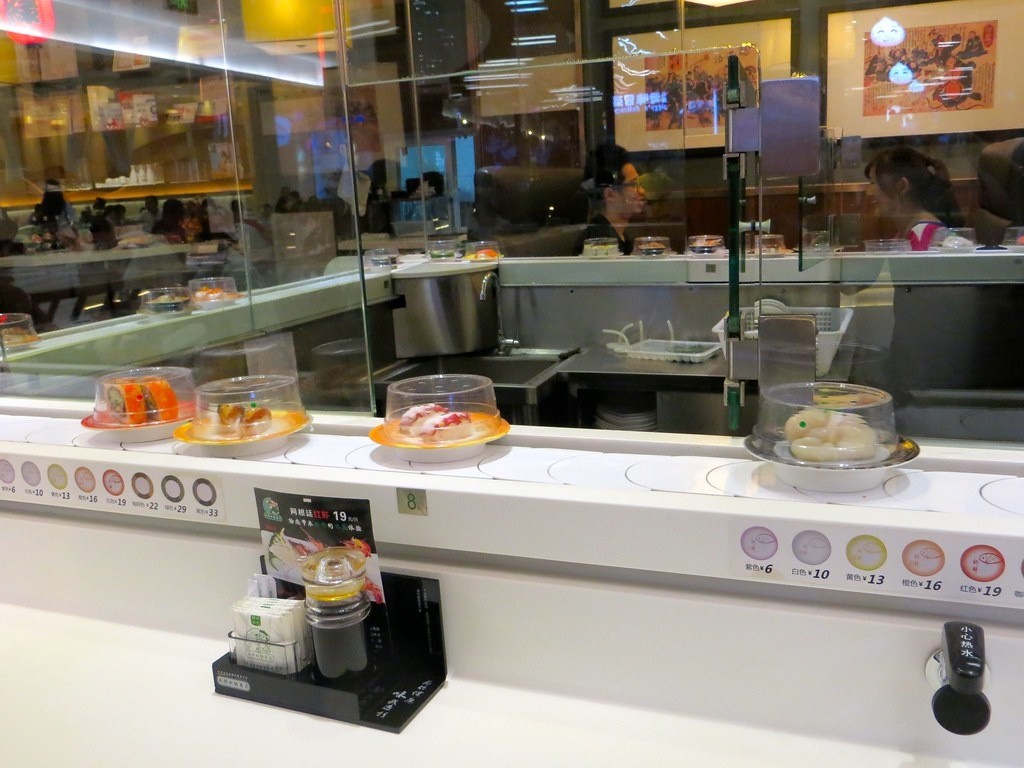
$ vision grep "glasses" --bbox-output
[609,180,642,191]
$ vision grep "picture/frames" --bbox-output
[818,2,1023,149]
[607,7,806,164]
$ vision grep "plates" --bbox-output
[998,244,1024,252]
[630,251,678,257]
[81,401,207,443]
[746,432,922,494]
[930,245,986,253]
[0,336,42,354]
[366,262,405,271]
[173,411,312,455]
[746,249,793,258]
[461,253,504,263]
[138,306,200,320]
[370,413,510,462]
[794,246,845,254]
[579,252,624,259]
[684,249,729,258]
[192,294,247,310]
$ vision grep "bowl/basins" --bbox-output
[751,389,901,464]
[189,277,238,300]
[428,240,459,258]
[633,236,671,255]
[686,235,725,254]
[385,373,499,443]
[422,253,462,261]
[1003,227,1024,245]
[466,240,501,259]
[196,374,307,440]
[1,312,38,346]
[137,285,195,312]
[87,367,200,426]
[810,232,840,246]
[755,235,786,252]
[863,239,913,254]
[934,227,975,246]
[583,238,620,256]
[365,249,399,265]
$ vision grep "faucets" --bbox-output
[478,270,522,356]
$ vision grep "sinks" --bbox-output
[358,340,590,407]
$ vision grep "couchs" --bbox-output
[957,133,1024,250]
[475,152,597,260]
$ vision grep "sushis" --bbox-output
[399,403,474,442]
[372,249,496,266]
[104,374,179,424]
[194,285,225,301]
[212,403,271,438]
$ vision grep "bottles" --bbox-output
[298,546,373,686]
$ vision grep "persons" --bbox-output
[571,143,648,258]
[0,126,496,322]
[836,125,1018,405]
[866,145,952,242]
